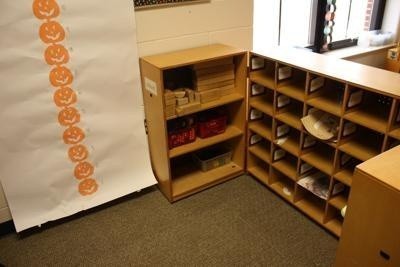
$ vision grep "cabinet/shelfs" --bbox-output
[139,43,248,203]
[247,45,400,238]
[334,146,400,267]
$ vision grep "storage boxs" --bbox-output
[195,112,227,138]
[168,121,197,149]
[193,149,232,173]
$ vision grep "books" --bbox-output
[160,52,236,121]
